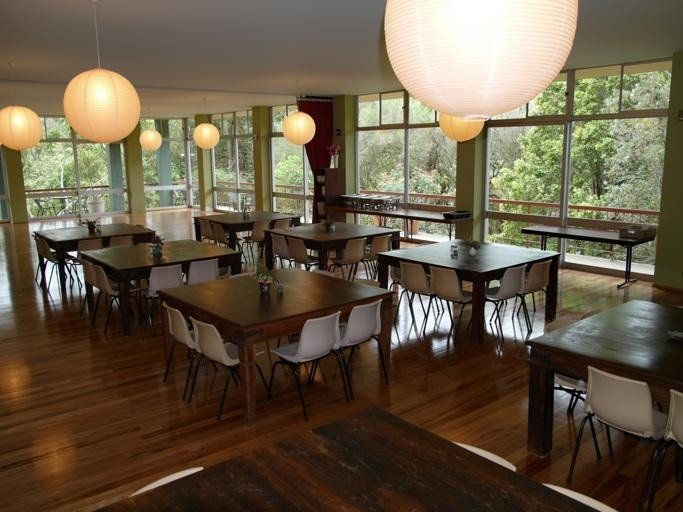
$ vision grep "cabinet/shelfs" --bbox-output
[312,170,346,224]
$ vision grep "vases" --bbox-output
[329,154,339,169]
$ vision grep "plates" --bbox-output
[665,328,682,342]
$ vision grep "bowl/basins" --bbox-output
[86,200,105,214]
[441,209,471,218]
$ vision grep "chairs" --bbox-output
[482,255,554,334]
[161,299,219,400]
[78,258,145,335]
[138,257,219,329]
[269,233,319,271]
[32,234,82,291]
[395,260,476,346]
[199,216,291,265]
[76,235,135,266]
[188,316,269,422]
[313,297,392,390]
[328,230,395,283]
[266,308,351,421]
[566,365,683,512]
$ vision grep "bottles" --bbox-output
[450,244,458,257]
[276,285,283,296]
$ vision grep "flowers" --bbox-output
[326,142,342,155]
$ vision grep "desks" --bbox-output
[32,221,156,298]
[351,206,476,245]
[520,222,658,290]
[188,207,302,255]
[156,268,397,424]
[76,241,244,336]
[34,402,598,511]
[262,218,405,271]
[518,298,683,457]
[375,237,563,329]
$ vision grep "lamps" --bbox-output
[127,1,161,152]
[437,110,487,145]
[59,0,143,147]
[178,0,222,152]
[379,0,580,117]
[269,0,320,145]
[0,0,45,154]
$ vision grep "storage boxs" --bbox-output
[338,193,400,210]
